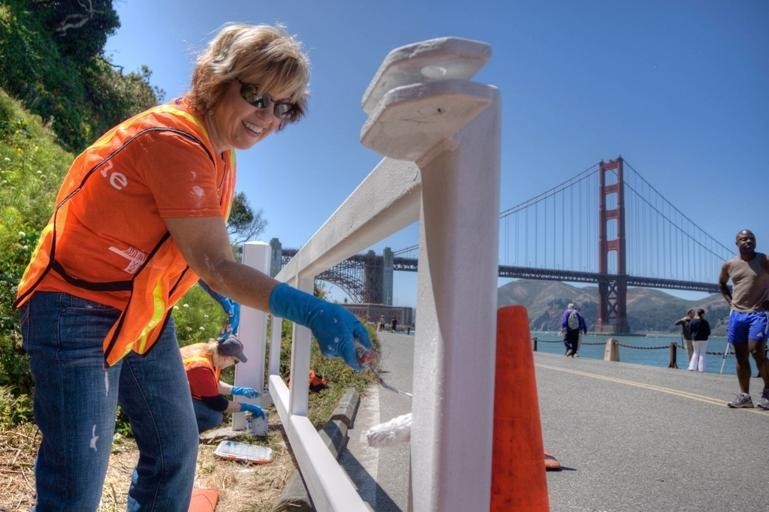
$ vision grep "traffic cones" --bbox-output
[492,303,556,512]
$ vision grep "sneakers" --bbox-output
[727,392,754,409]
[566,349,579,358]
[757,388,769,410]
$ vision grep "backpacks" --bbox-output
[568,309,580,330]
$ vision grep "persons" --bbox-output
[689,307,711,372]
[560,302,588,359]
[177,335,266,435]
[675,309,694,363]
[12,21,370,512]
[720,229,769,411]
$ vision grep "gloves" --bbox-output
[198,278,241,334]
[267,281,373,373]
[230,385,264,400]
[239,401,266,422]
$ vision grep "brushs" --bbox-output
[355,340,412,448]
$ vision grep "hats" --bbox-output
[217,338,248,363]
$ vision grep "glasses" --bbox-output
[227,356,240,364]
[233,76,298,122]
